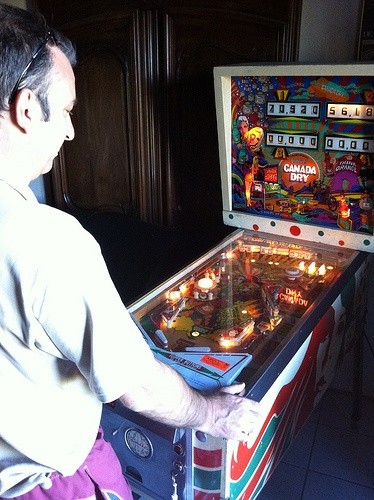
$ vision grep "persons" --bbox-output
[0,4,272,500]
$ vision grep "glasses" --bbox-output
[8,25,58,104]
